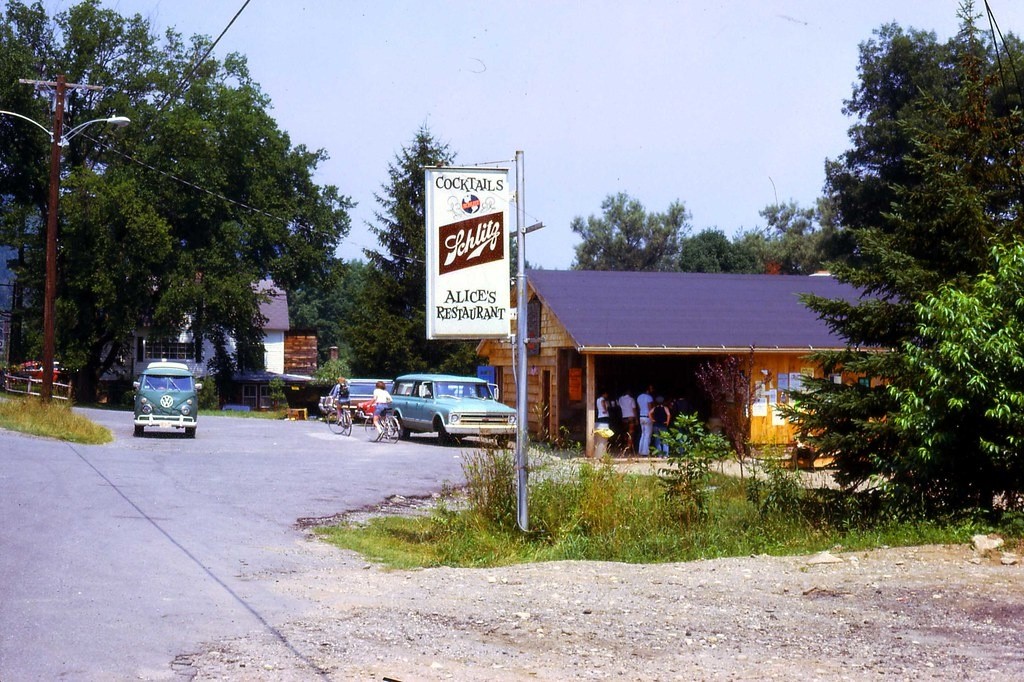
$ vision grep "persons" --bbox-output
[369,381,393,439]
[672,396,691,456]
[637,384,653,457]
[650,396,670,459]
[332,377,352,423]
[597,392,609,422]
[619,388,638,458]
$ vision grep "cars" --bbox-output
[133,362,202,439]
[16,360,62,382]
[383,374,517,449]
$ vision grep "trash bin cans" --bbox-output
[594,422,609,458]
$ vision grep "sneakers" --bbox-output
[335,417,349,429]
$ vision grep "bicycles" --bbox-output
[325,396,353,436]
[364,405,401,444]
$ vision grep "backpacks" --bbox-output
[338,383,349,398]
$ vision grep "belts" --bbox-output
[377,403,388,405]
[640,415,650,418]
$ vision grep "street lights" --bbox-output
[0,110,132,403]
